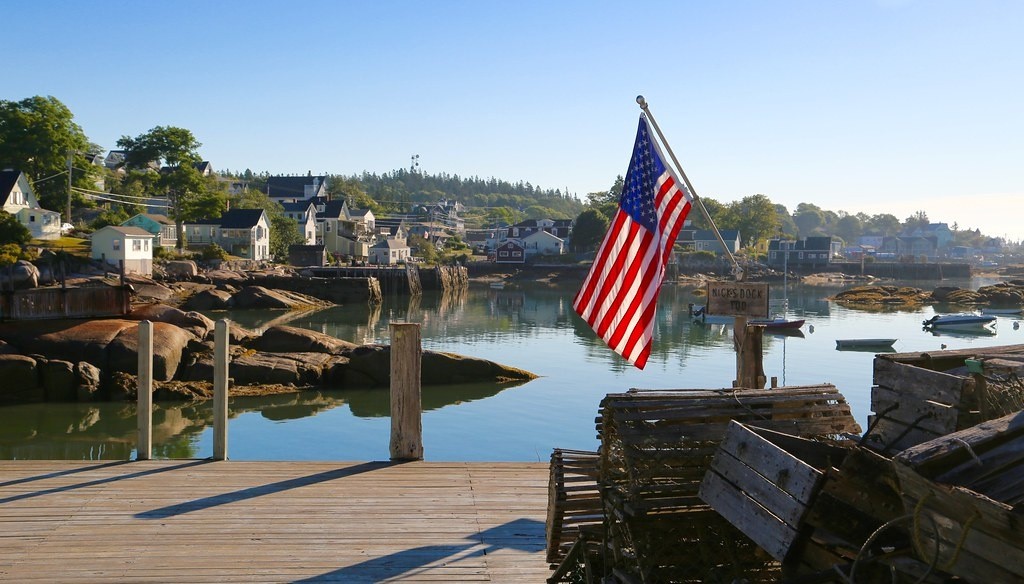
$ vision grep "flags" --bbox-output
[572,112,693,370]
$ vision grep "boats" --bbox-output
[982,307,1021,315]
[925,314,999,329]
[835,336,897,348]
[685,302,807,331]
